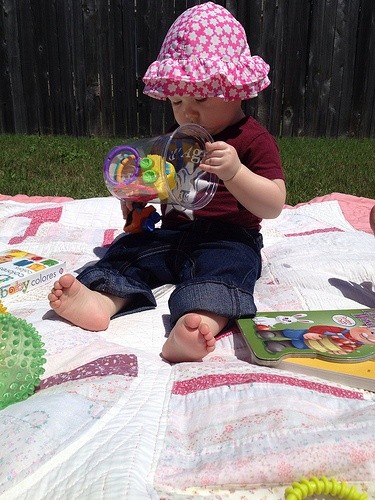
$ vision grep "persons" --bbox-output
[47,1,287,365]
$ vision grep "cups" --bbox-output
[102,123,218,210]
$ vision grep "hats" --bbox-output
[142,2,271,101]
